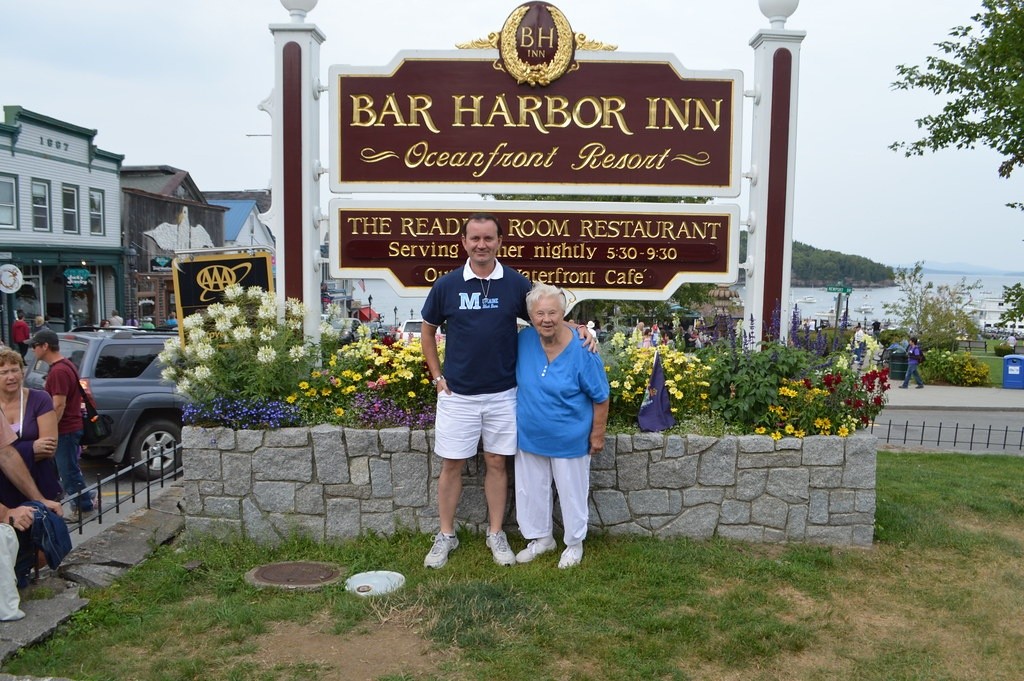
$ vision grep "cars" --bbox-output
[321,279,447,347]
[651,304,702,326]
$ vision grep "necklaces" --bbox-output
[479,277,490,298]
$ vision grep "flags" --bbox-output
[638,353,677,432]
[358,279,365,291]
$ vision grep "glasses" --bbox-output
[31,345,45,349]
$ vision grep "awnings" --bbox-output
[359,308,379,321]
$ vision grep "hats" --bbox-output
[23,330,59,346]
[586,321,595,328]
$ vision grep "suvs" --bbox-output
[21,326,200,482]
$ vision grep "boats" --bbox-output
[815,307,851,318]
[860,303,875,313]
[953,291,1024,328]
[798,296,817,303]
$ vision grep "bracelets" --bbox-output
[576,324,585,331]
[432,375,443,386]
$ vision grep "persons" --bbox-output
[100,319,110,328]
[420,212,598,570]
[1006,333,1017,346]
[13,308,30,367]
[886,333,911,353]
[632,320,714,351]
[108,309,123,326]
[899,336,924,388]
[23,331,100,526]
[35,316,50,336]
[0,341,64,621]
[516,283,610,568]
[126,312,178,329]
[586,320,600,345]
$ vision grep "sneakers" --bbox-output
[63,512,96,523]
[558,547,583,569]
[486,527,515,566]
[424,530,459,569]
[516,539,556,563]
[93,491,102,510]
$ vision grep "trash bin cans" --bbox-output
[888,347,909,380]
[1002,355,1024,389]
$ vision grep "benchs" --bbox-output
[958,340,988,354]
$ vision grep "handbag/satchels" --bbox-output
[913,347,926,364]
[81,414,115,441]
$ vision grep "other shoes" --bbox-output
[899,385,908,389]
[915,385,924,389]
[23,360,27,366]
